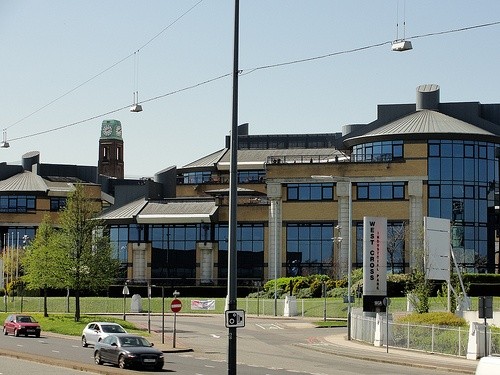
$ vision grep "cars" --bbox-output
[94,334,165,371]
[2,314,42,338]
[81,322,130,348]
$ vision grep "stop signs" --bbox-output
[171,299,183,313]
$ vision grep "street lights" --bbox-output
[331,225,344,280]
